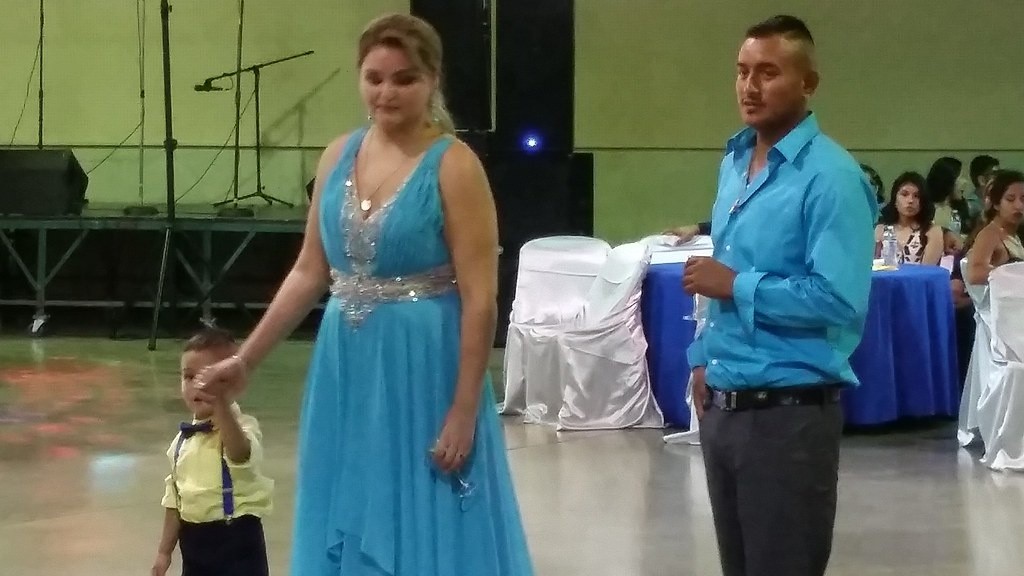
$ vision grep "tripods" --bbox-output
[204,50,314,209]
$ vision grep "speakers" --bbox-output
[0,149,89,216]
[406,0,574,154]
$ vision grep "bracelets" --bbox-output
[233,354,252,374]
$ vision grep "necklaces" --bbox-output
[992,221,1021,246]
[360,128,403,211]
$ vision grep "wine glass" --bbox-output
[682,255,712,322]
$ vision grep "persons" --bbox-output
[193,13,534,576]
[686,17,878,576]
[150,329,274,576]
[661,220,712,246]
[861,155,1024,307]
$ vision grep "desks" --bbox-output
[640,263,959,430]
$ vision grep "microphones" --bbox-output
[194,85,224,91]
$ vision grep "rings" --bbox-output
[457,453,464,458]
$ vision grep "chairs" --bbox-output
[498,237,612,415]
[526,244,664,430]
[976,262,1024,470]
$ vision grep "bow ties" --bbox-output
[180,420,213,439]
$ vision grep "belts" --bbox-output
[712,382,840,411]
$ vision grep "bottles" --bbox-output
[949,209,962,236]
[882,226,897,265]
